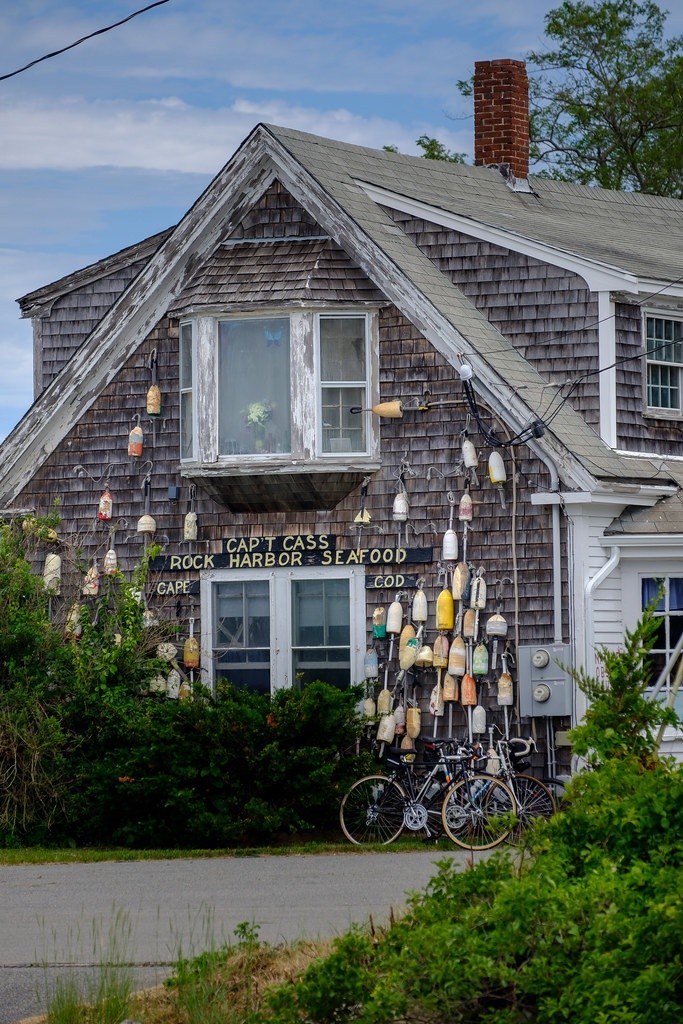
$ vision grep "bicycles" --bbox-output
[339,722,571,852]
